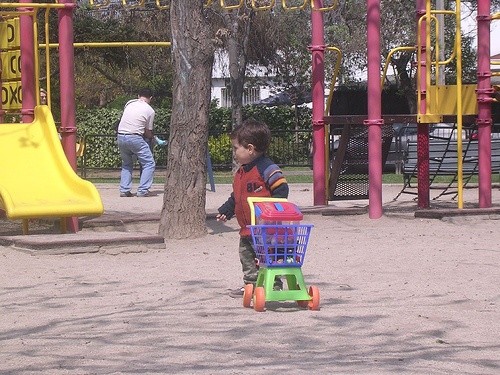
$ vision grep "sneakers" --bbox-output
[120,192,133,197]
[228,288,254,298]
[138,192,156,196]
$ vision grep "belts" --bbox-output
[118,132,138,135]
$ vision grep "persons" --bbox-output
[117,88,157,197]
[216,118,289,298]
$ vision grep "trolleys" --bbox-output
[242,196,321,314]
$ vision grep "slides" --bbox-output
[0,104,105,217]
